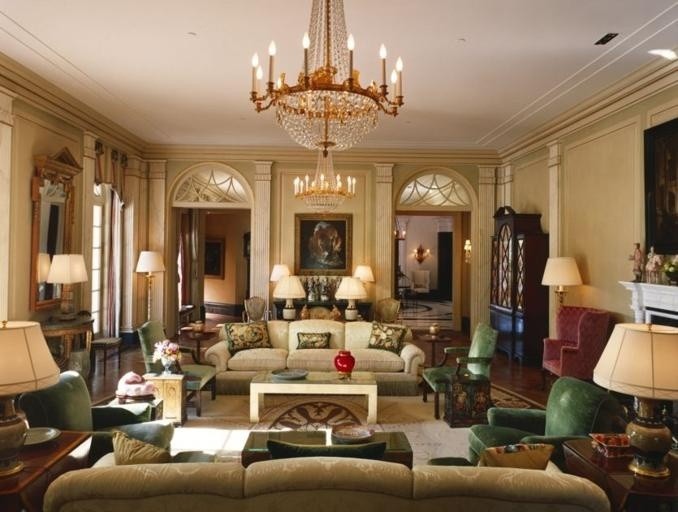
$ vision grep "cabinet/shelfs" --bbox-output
[488,210,548,368]
[146,372,187,427]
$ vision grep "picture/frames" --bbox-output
[292,212,351,278]
[205,235,225,281]
[641,118,677,254]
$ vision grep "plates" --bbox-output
[24,426,61,447]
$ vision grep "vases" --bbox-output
[335,349,354,380]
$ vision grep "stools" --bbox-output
[91,336,122,365]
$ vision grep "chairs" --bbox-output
[421,322,500,422]
[135,321,214,417]
[464,376,621,463]
[419,334,449,367]
[411,270,431,296]
[536,304,616,387]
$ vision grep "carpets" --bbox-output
[90,381,545,429]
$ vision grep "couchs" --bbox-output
[40,463,614,510]
[17,374,154,464]
[203,320,426,397]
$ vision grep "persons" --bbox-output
[644,245,662,283]
[628,242,643,284]
[298,276,342,303]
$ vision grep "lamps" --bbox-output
[354,266,374,281]
[271,264,290,282]
[591,320,677,478]
[270,277,306,321]
[37,252,51,299]
[248,1,406,208]
[53,250,89,317]
[412,242,431,263]
[134,249,166,321]
[336,276,367,320]
[0,316,59,476]
[541,257,582,311]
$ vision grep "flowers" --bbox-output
[152,340,179,366]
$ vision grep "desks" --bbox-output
[43,316,94,364]
[565,440,677,511]
[0,429,94,509]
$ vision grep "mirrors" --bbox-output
[26,146,89,311]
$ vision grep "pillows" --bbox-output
[266,439,387,459]
[368,319,404,354]
[221,321,271,352]
[295,331,332,348]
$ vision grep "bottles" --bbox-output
[335,351,354,382]
